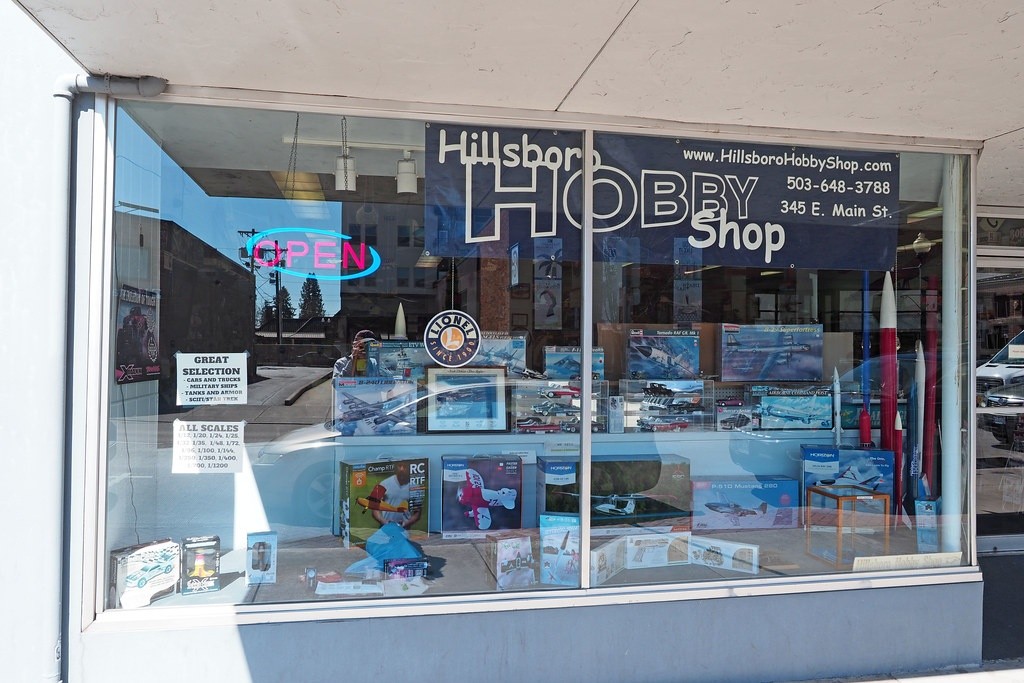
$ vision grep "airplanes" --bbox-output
[468,345,524,372]
[342,392,415,429]
[355,484,409,514]
[704,491,767,527]
[521,367,548,381]
[725,326,810,379]
[767,394,831,425]
[813,466,883,491]
[636,346,695,377]
[555,356,580,373]
[456,469,518,531]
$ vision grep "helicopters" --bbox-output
[550,489,679,516]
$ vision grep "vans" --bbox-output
[976,329,1024,403]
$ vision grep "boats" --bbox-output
[396,344,423,378]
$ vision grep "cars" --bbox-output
[728,354,941,496]
[124,562,174,588]
[514,371,751,433]
[976,381,1023,444]
[250,374,662,527]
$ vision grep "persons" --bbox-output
[366,461,425,535]
[331,331,397,467]
